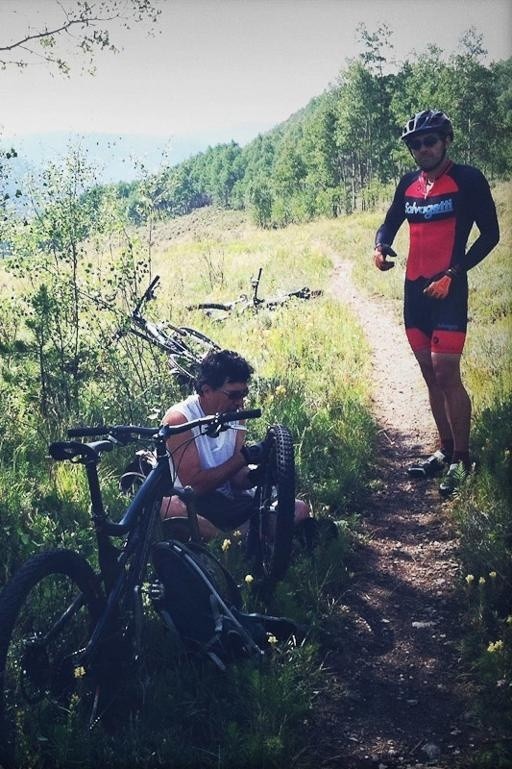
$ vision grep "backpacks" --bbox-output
[150,539,298,672]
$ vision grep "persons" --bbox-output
[371,106,501,499]
[149,346,311,551]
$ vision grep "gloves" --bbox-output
[421,270,455,300]
[374,242,398,271]
[248,465,265,485]
[239,427,277,464]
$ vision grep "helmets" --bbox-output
[401,109,452,140]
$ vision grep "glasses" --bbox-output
[215,386,249,401]
[407,135,437,150]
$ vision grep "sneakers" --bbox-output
[438,462,469,495]
[406,447,452,478]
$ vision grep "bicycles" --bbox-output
[0,407,268,769]
[105,273,223,397]
[186,266,321,329]
